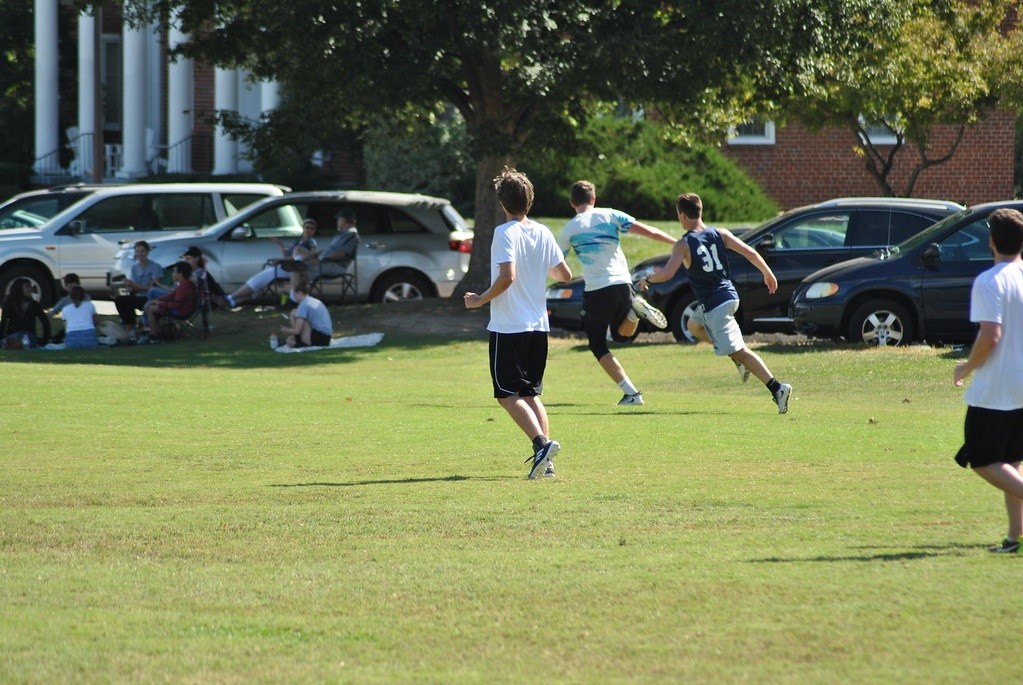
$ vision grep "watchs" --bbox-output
[645,273,650,284]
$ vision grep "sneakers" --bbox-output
[631,297,668,329]
[545,459,556,477]
[617,391,644,406]
[772,382,793,415]
[524,439,561,479]
[736,362,752,383]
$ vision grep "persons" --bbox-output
[0,279,50,350]
[115,238,162,330]
[147,262,198,343]
[211,219,318,311]
[45,273,89,342]
[280,281,333,348]
[137,246,208,335]
[277,207,359,315]
[636,193,791,413]
[60,286,98,348]
[557,181,677,405]
[954,208,1023,554]
[464,165,572,480]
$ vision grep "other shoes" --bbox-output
[989,536,1022,553]
[147,335,161,344]
[212,292,232,311]
[136,329,150,339]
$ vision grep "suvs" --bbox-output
[106,191,474,320]
[0,182,317,322]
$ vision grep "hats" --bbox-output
[180,247,202,259]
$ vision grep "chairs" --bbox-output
[262,250,322,311]
[309,241,358,307]
[120,264,213,343]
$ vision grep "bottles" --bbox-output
[270,333,278,349]
[22,335,29,350]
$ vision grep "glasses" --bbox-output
[304,225,317,232]
[182,255,196,261]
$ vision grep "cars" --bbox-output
[790,199,1022,347]
[543,228,852,335]
[641,197,968,332]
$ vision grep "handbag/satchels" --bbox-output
[5,329,37,350]
